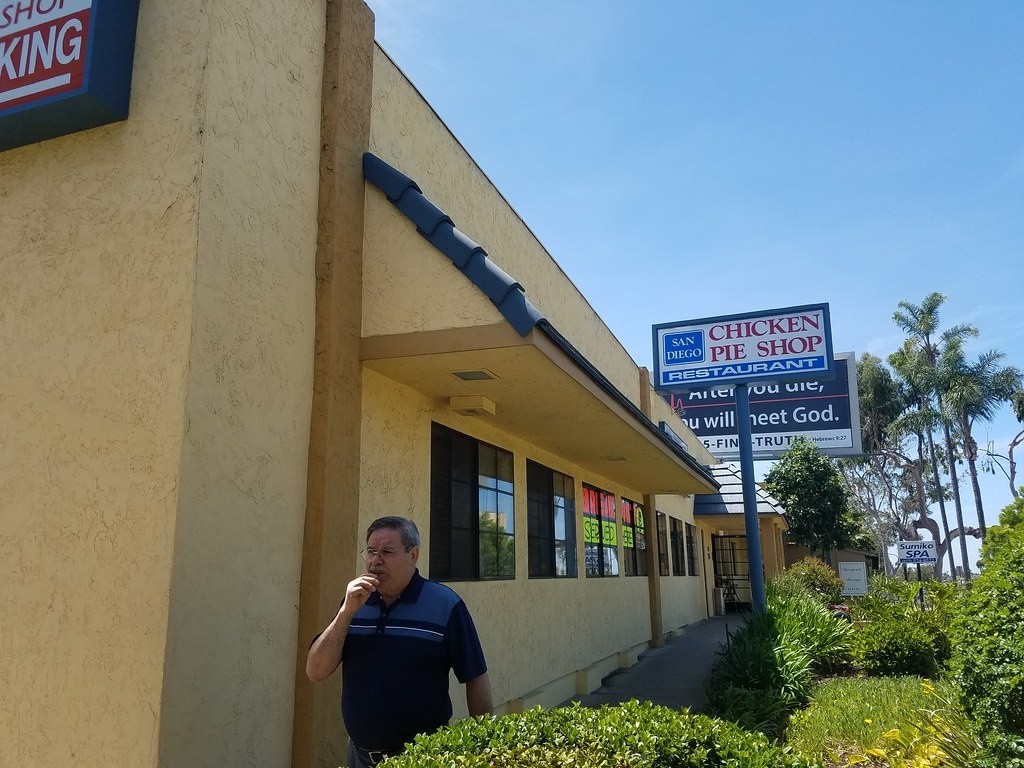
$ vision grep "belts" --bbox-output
[352,740,415,765]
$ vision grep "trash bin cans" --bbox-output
[714,587,726,616]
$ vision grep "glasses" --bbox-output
[360,544,411,562]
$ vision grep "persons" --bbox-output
[306,516,494,768]
